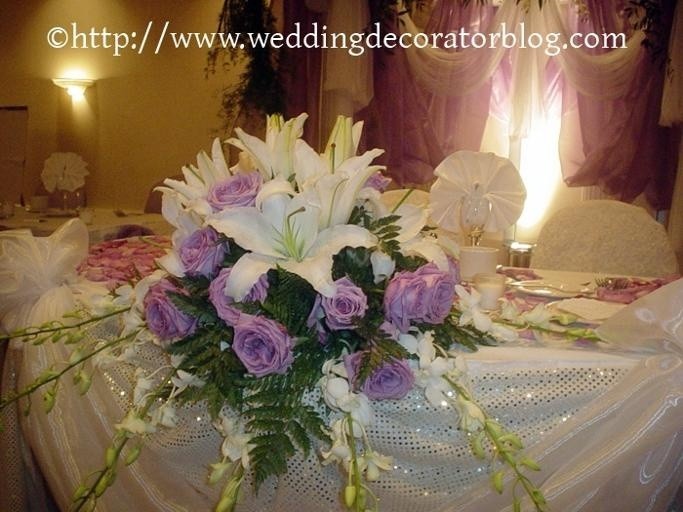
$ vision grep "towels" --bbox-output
[40,149,90,193]
[428,147,530,238]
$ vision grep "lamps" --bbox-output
[51,76,94,98]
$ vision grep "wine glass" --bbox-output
[459,196,494,247]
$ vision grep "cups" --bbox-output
[471,272,508,311]
[77,206,96,226]
[458,244,499,282]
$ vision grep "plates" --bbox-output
[517,280,586,297]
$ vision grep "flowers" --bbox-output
[0,110,607,511]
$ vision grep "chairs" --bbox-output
[375,187,465,248]
[530,198,679,277]
[34,175,86,209]
[142,174,187,215]
